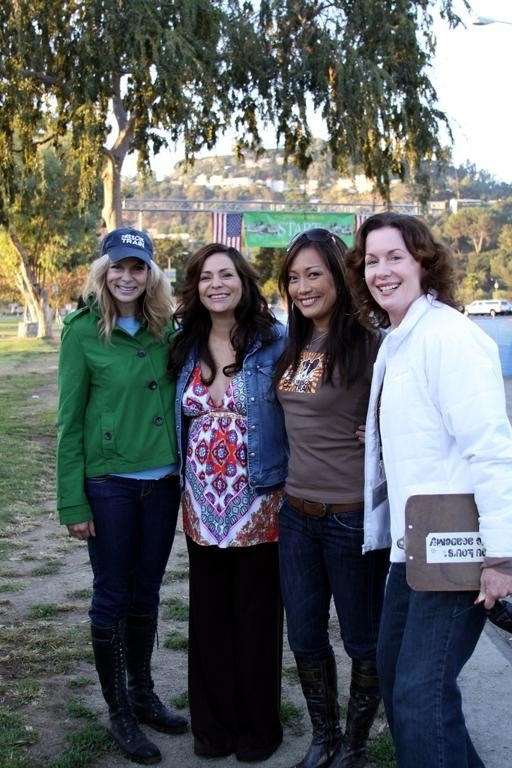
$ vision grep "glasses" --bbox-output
[285,229,340,253]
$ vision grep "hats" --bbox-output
[101,226,153,270]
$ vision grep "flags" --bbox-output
[211,211,243,252]
[355,215,372,233]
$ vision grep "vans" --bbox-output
[464,299,511,316]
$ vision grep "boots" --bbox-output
[88,620,162,763]
[328,660,382,768]
[288,652,339,768]
[119,615,188,735]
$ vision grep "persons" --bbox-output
[166,242,293,763]
[53,226,188,765]
[343,210,512,767]
[267,226,389,768]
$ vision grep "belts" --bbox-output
[286,493,364,517]
